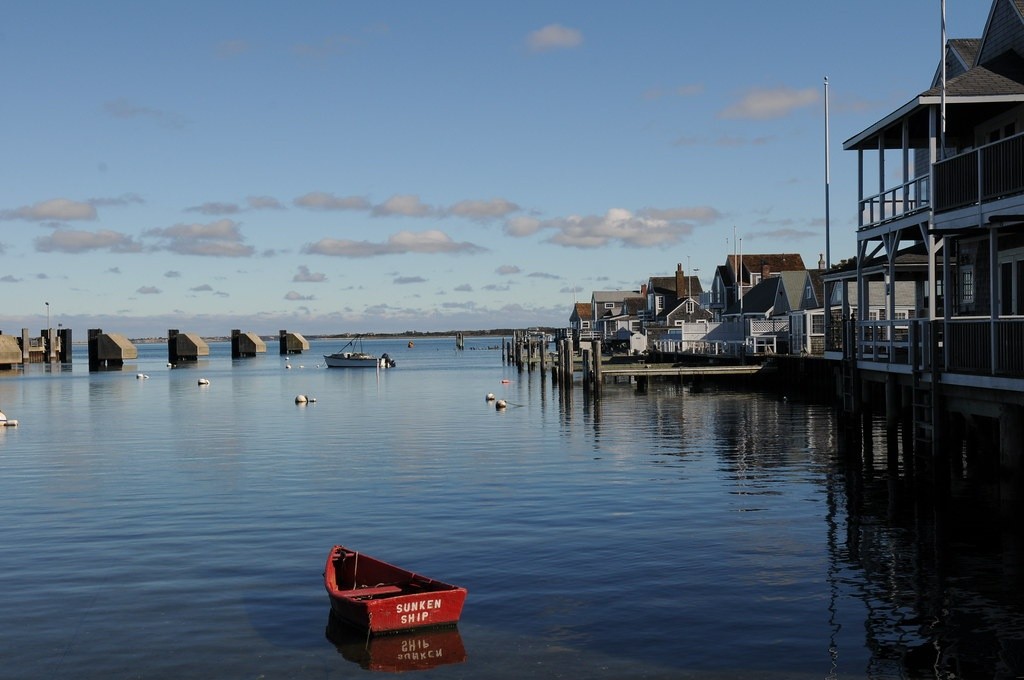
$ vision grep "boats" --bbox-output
[323,332,397,368]
[324,544,469,636]
[326,608,467,672]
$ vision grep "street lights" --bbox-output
[45,302,49,340]
